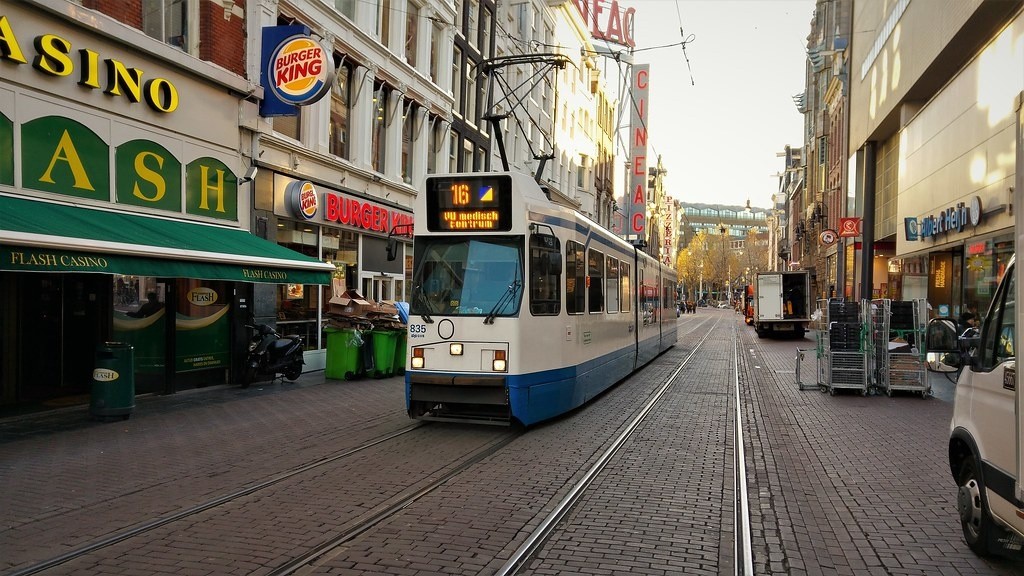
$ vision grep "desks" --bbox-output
[276,317,328,348]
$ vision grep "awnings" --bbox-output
[0,190,337,287]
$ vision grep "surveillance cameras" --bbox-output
[239,167,258,184]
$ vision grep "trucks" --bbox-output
[748,270,810,339]
[741,284,754,324]
[925,88,1024,557]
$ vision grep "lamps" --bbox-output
[303,225,312,232]
[793,202,828,239]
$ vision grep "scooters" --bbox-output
[240,319,306,387]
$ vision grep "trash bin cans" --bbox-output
[322,327,369,379]
[395,329,408,375]
[88,341,138,419]
[372,329,401,375]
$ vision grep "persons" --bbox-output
[955,311,982,339]
[677,300,698,317]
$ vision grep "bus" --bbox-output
[403,51,681,431]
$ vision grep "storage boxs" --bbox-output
[830,301,921,384]
[328,288,399,318]
